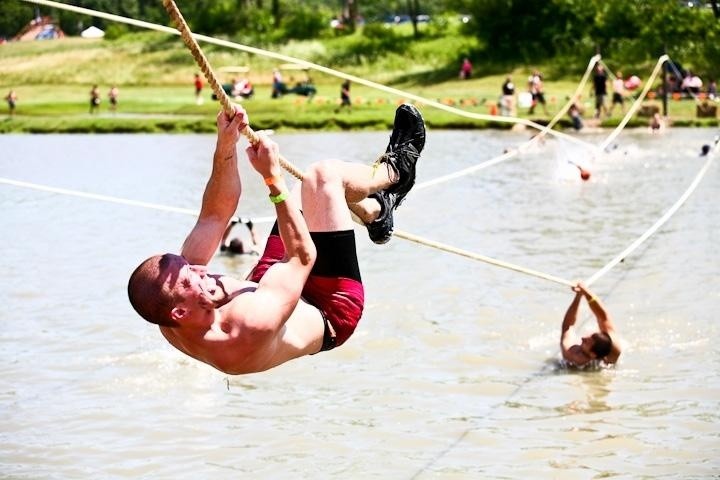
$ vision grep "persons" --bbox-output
[108,86,119,112]
[126,102,425,376]
[330,11,364,35]
[581,169,591,181]
[89,83,102,115]
[501,62,720,132]
[460,58,473,79]
[6,88,17,119]
[220,215,260,257]
[699,144,711,156]
[192,67,352,114]
[560,281,621,367]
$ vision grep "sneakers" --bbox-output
[380,104,425,184]
[366,184,414,244]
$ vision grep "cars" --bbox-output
[382,11,473,23]
[331,13,367,30]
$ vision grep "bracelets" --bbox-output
[264,172,286,186]
[587,295,598,304]
[269,187,290,204]
